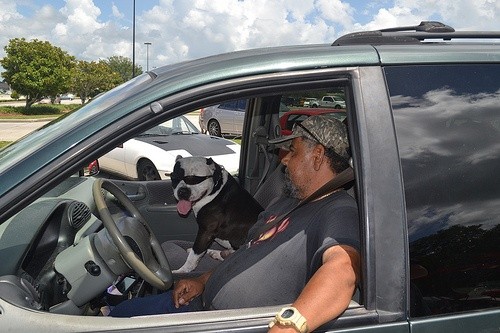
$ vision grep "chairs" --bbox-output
[161,164,301,272]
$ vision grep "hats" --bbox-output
[268,114,351,161]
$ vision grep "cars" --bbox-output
[199,96,247,137]
[98,115,241,182]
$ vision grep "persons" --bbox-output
[107,114,361,333]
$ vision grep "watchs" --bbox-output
[268,307,307,333]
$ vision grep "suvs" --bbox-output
[1,21,500,333]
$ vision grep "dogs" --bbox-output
[171,155,266,274]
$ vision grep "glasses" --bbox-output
[170,172,223,185]
[292,120,328,151]
